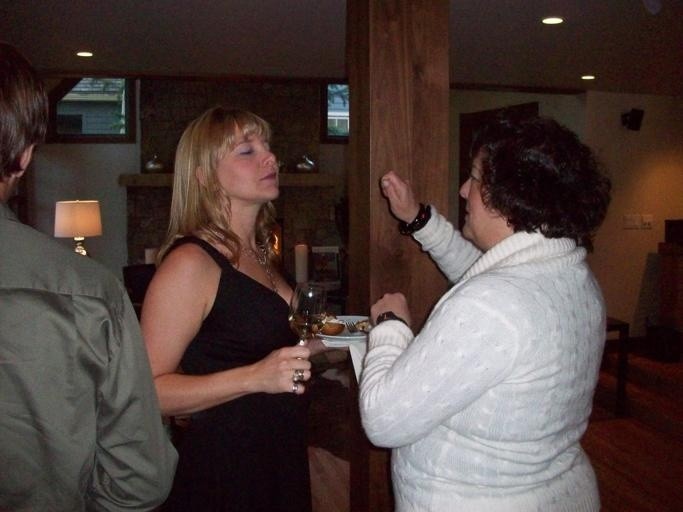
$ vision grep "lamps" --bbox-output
[53,199,103,257]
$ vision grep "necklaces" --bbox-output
[237,245,281,293]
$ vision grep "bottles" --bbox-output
[144,152,169,173]
[295,155,317,173]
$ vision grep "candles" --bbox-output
[146,248,157,264]
[295,244,308,284]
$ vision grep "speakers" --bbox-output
[627,108,644,131]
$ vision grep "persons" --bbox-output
[351,109,617,510]
[0,44,184,511]
[134,100,351,510]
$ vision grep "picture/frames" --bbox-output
[458,102,540,230]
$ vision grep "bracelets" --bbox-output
[398,203,433,238]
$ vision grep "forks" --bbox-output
[345,320,370,337]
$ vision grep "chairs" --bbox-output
[123,263,156,320]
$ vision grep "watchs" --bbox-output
[374,310,408,324]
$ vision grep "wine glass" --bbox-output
[287,283,328,380]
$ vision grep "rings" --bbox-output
[291,384,300,395]
[292,368,306,382]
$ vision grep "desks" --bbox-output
[604,316,629,410]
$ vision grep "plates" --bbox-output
[287,314,374,349]
